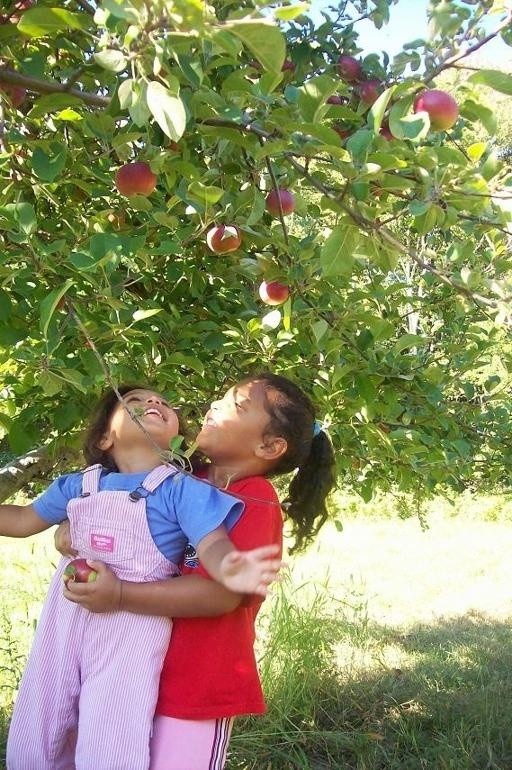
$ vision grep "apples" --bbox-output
[2,1,34,24]
[207,224,243,255]
[62,558,99,590]
[114,163,158,198]
[266,188,294,217]
[1,82,27,106]
[168,140,177,151]
[248,61,262,79]
[281,60,296,70]
[414,88,459,134]
[326,53,395,141]
[259,280,290,306]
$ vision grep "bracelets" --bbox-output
[119,580,123,612]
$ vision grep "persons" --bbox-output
[58,373,337,770]
[0,386,287,770]
